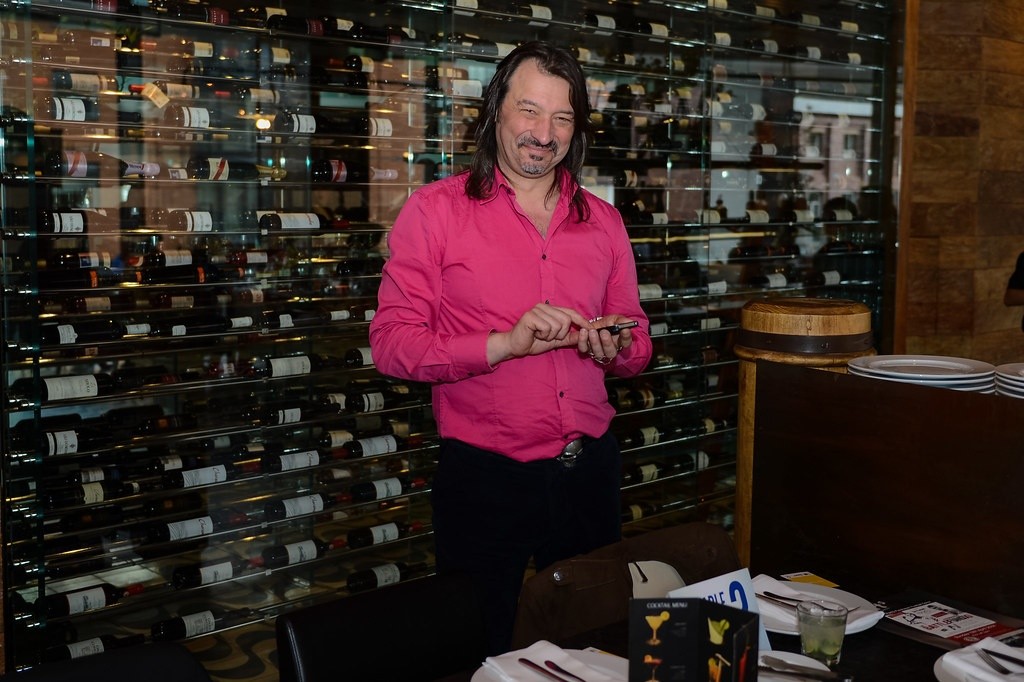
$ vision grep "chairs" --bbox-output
[274,570,514,682]
[18,643,212,682]
[520,523,743,643]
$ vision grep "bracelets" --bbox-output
[591,352,620,365]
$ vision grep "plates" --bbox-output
[471,649,629,682]
[934,647,1024,682]
[759,581,878,635]
[848,355,1024,399]
[758,650,831,682]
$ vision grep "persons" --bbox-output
[369,40,653,672]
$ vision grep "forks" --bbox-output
[976,649,1024,676]
[764,591,859,613]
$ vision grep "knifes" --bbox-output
[981,648,1024,667]
[755,593,798,611]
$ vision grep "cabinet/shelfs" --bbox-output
[1,0,901,682]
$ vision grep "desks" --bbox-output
[558,560,1024,682]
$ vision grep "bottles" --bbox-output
[1,0,903,682]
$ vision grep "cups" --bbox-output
[797,600,847,666]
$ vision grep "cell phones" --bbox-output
[596,320,639,336]
